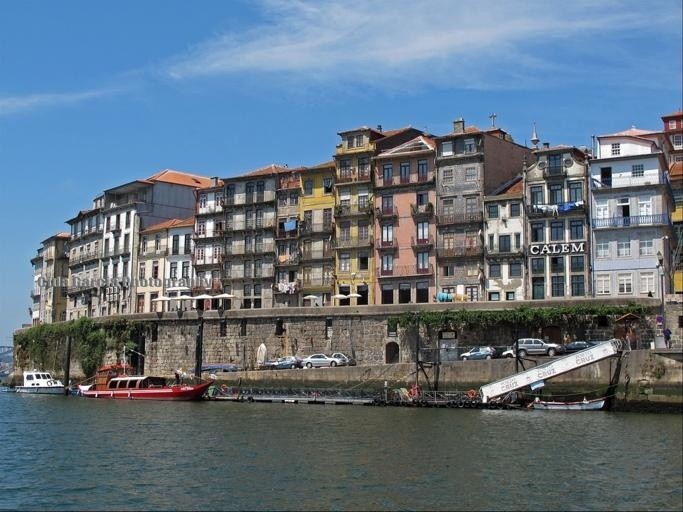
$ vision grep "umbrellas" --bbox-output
[303,295,318,300]
[331,294,362,299]
[152,294,236,311]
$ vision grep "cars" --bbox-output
[264,356,303,371]
[329,352,356,367]
[564,340,594,352]
[460,346,497,361]
[501,347,515,359]
[513,338,566,358]
[305,353,344,369]
[191,364,237,373]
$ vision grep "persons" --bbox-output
[656,314,663,329]
[662,325,672,348]
[648,291,654,297]
[627,327,637,350]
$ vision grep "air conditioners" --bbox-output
[106,287,120,302]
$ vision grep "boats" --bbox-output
[79,364,214,402]
[15,371,65,393]
[533,398,607,411]
